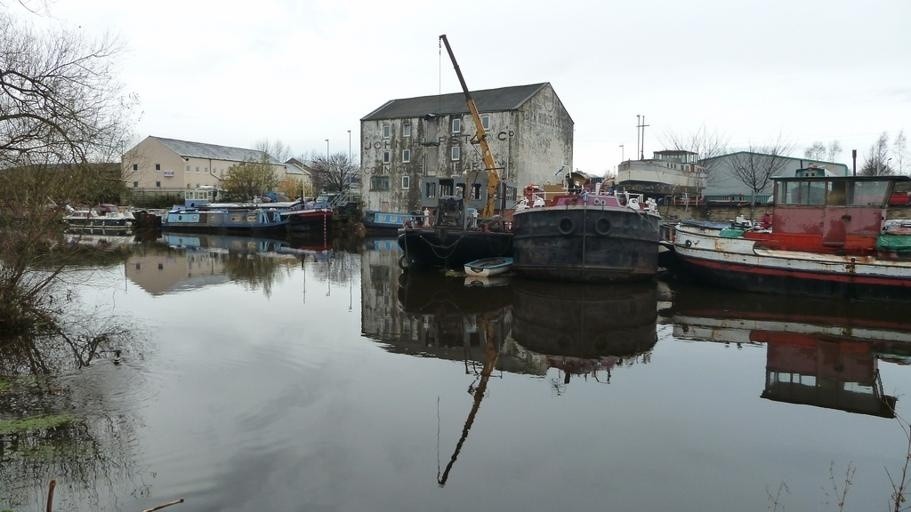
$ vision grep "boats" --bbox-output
[64,200,334,242]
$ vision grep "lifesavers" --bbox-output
[594,216,612,235]
[557,216,575,235]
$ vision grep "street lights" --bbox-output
[326,139,329,201]
[348,131,352,203]
